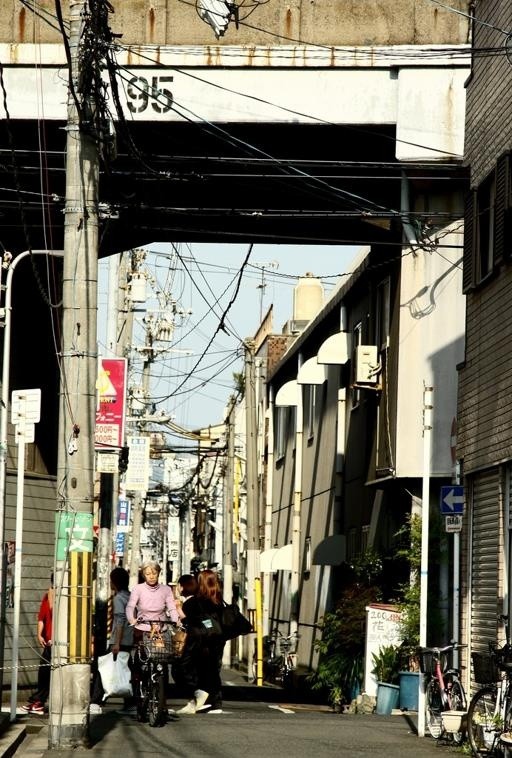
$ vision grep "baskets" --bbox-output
[472,652,502,684]
[138,641,183,659]
[417,647,444,672]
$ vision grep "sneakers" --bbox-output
[116,706,138,715]
[176,690,223,714]
[89,704,102,714]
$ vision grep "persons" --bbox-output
[18,571,54,716]
[89,559,225,721]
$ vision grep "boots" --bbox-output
[20,700,45,715]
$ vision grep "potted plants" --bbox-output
[305,546,390,707]
[397,647,420,709]
[372,646,400,715]
[480,715,502,743]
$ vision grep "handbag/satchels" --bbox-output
[143,625,174,659]
[220,603,252,640]
[98,651,134,701]
[194,614,222,639]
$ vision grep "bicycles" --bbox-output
[415,643,467,747]
[251,628,299,684]
[467,641,512,758]
[129,618,180,727]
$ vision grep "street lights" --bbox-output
[121,415,170,425]
[3,249,66,539]
